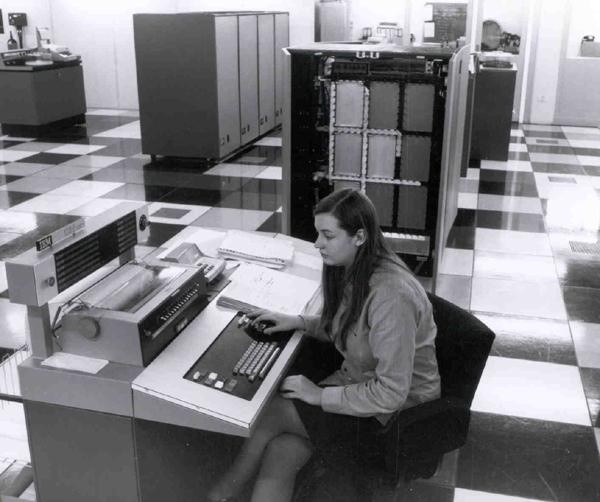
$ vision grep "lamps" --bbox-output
[316,289,495,502]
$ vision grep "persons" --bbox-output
[206,185,440,502]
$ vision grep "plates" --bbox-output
[132,10,288,164]
